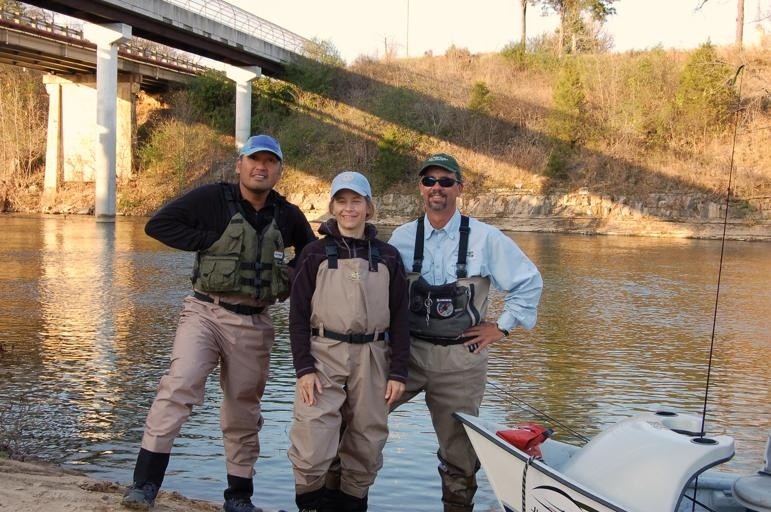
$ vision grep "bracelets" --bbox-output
[495,320,509,336]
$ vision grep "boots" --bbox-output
[120,447,171,510]
[223,474,263,512]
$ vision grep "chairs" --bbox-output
[731,429,771,512]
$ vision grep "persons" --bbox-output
[121,133,315,511]
[288,171,412,511]
[385,151,544,511]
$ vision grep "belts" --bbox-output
[312,328,385,344]
[193,290,265,315]
[409,334,478,346]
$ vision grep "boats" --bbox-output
[448,402,770,511]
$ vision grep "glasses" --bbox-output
[421,176,461,187]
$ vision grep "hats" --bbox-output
[330,172,371,202]
[239,135,283,161]
[418,153,459,176]
